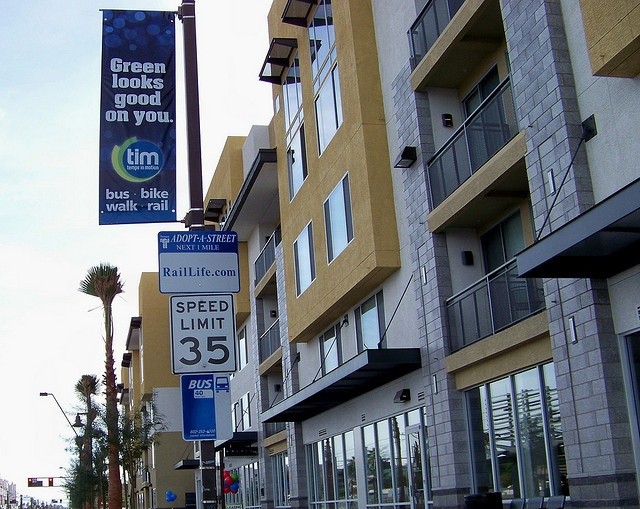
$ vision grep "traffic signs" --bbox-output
[169,293,239,375]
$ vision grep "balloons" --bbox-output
[223,469,240,496]
[165,490,178,502]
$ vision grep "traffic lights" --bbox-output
[49,478,53,486]
[28,478,32,487]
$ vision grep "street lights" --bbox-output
[40,393,78,437]
